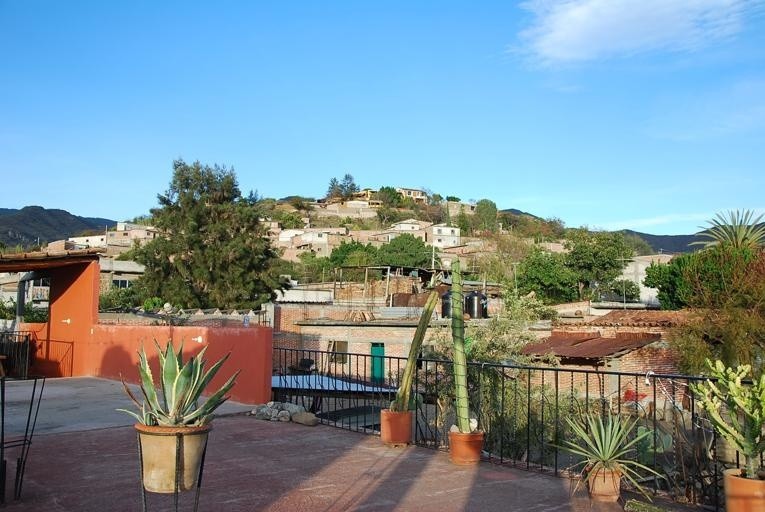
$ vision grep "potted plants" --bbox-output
[379,291,440,449]
[114,335,241,494]
[544,408,668,509]
[446,256,485,467]
[687,358,765,512]
